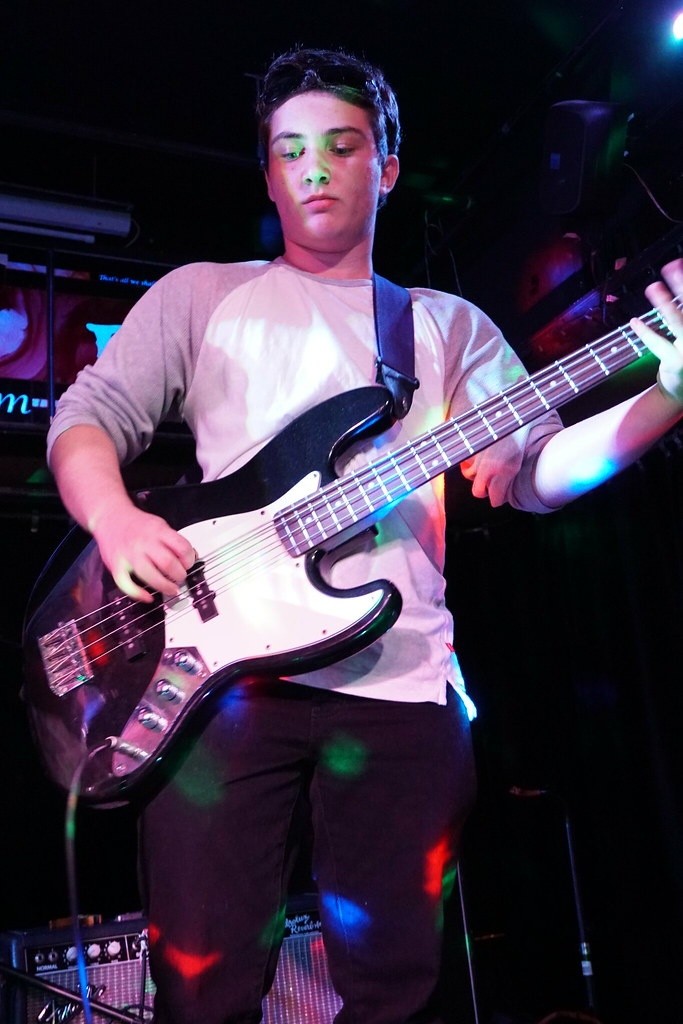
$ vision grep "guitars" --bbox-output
[19,293,682,818]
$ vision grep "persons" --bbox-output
[47,43,683,1024]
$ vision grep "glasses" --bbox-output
[260,61,384,111]
